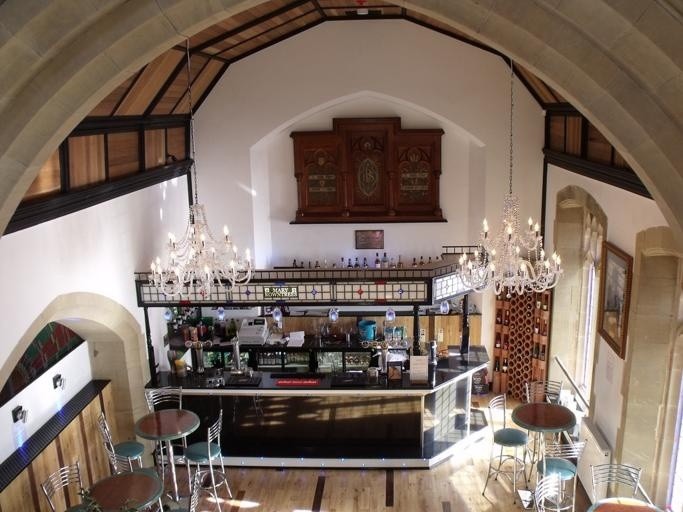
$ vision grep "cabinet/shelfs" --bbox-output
[168,331,409,371]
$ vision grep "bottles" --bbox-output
[189,318,239,343]
[502,358,507,372]
[367,368,378,383]
[494,337,544,360]
[317,352,342,367]
[291,252,441,269]
[258,351,309,365]
[459,298,463,313]
[495,361,499,372]
[204,352,249,370]
[495,311,546,336]
[345,354,370,365]
[496,287,547,310]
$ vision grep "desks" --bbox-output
[511,403,576,482]
[88,471,164,512]
[134,409,201,502]
[586,497,665,512]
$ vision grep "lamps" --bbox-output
[148,39,251,298]
[459,60,562,299]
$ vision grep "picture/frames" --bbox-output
[596,241,634,360]
[355,230,384,249]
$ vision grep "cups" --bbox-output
[427,357,436,385]
[175,364,192,377]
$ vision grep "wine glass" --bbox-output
[166,350,176,378]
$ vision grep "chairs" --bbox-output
[95,412,145,473]
[482,393,527,505]
[182,409,232,512]
[532,439,588,512]
[41,461,85,512]
[525,380,563,482]
[165,469,198,512]
[590,463,642,502]
[144,386,192,493]
[533,472,561,512]
